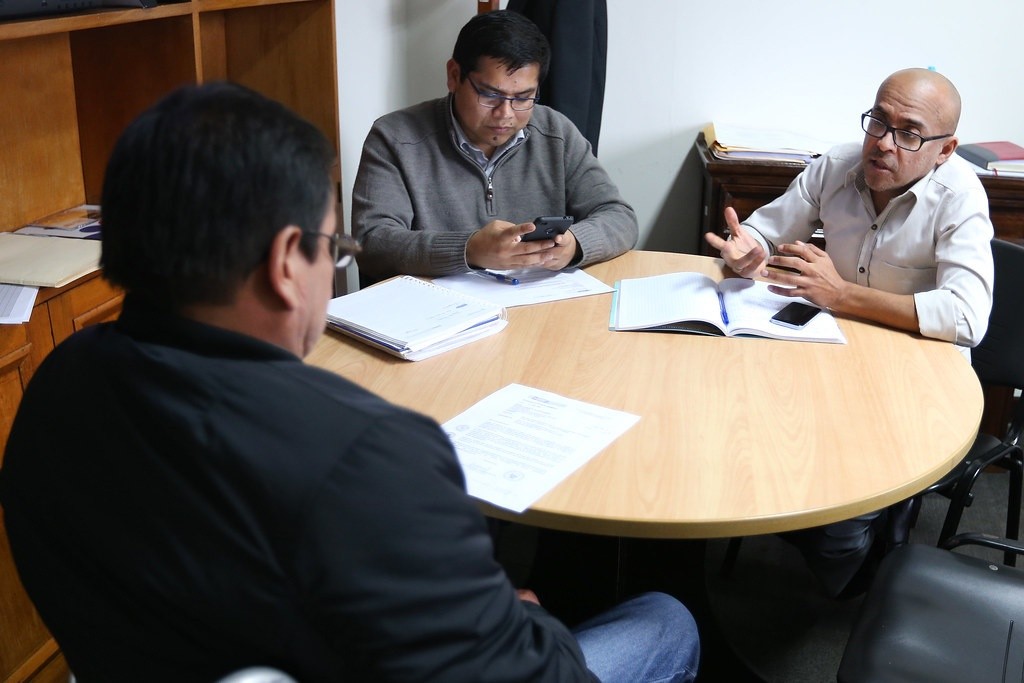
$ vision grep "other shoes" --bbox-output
[817,564,873,605]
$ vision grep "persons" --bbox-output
[351,10,639,291]
[0,79,700,683]
[705,67,995,613]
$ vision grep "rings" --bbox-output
[733,266,744,273]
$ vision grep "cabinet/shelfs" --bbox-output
[695,141,1024,259]
[0,0,349,683]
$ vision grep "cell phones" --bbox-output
[520,216,573,243]
[770,302,822,329]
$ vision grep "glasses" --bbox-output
[860,108,954,153]
[463,71,541,111]
[300,227,359,272]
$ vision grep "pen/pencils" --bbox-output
[717,291,730,325]
[479,270,519,285]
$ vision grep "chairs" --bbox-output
[722,238,1024,568]
[836,532,1024,683]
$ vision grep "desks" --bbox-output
[304,250,984,683]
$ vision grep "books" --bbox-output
[609,272,849,344]
[327,274,508,363]
[956,140,1024,173]
[0,230,103,289]
